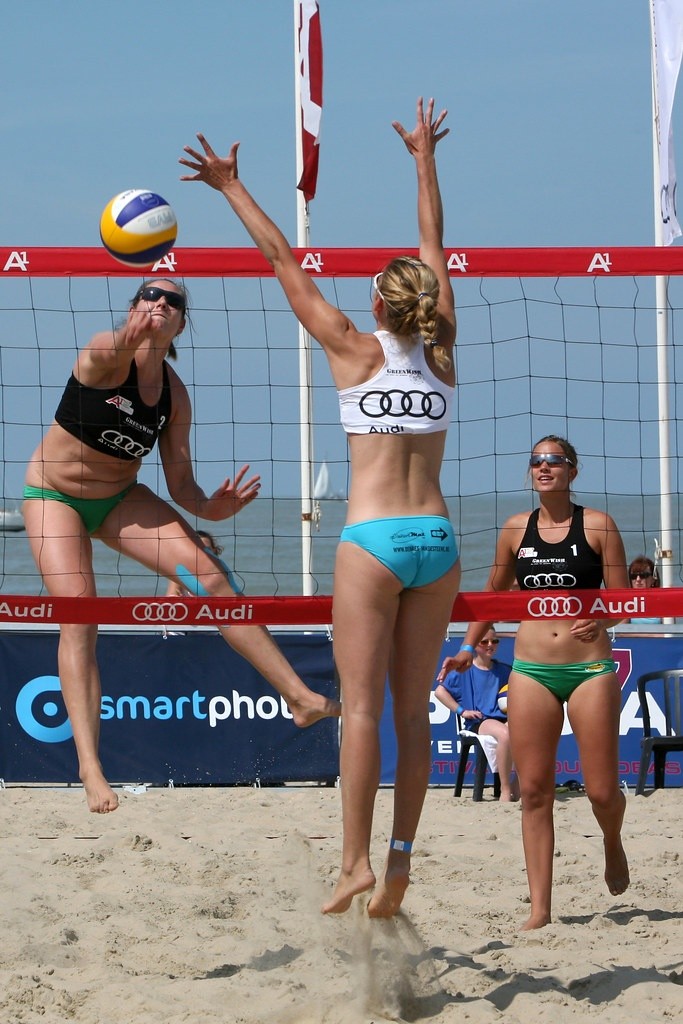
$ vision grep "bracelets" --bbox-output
[456,705,465,715]
[461,644,474,656]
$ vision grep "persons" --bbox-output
[436,433,632,930]
[167,530,242,597]
[435,625,522,801]
[20,278,340,815]
[177,94,463,915]
[621,555,661,624]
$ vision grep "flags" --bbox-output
[297,1,322,205]
[653,0,683,246]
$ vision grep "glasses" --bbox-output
[529,454,573,465]
[629,572,652,579]
[373,272,402,314]
[139,287,186,310]
[478,638,499,647]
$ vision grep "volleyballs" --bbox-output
[100,187,178,268]
[497,683,509,714]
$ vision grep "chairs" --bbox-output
[453,713,501,802]
[635,669,683,796]
